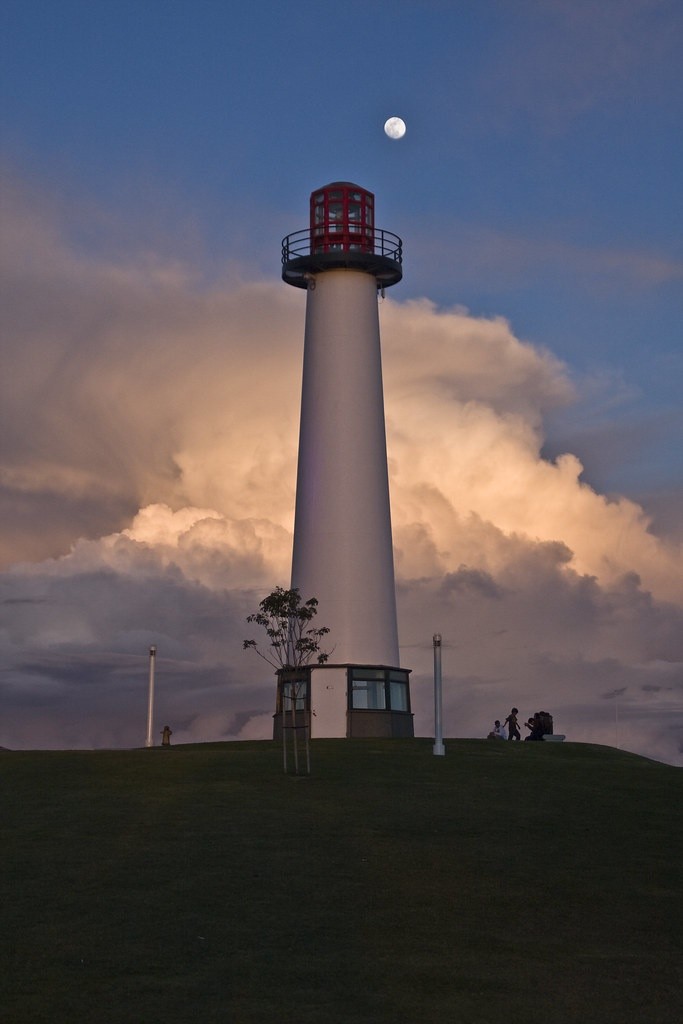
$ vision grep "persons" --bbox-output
[494,720,504,735]
[503,707,520,740]
[524,711,553,741]
